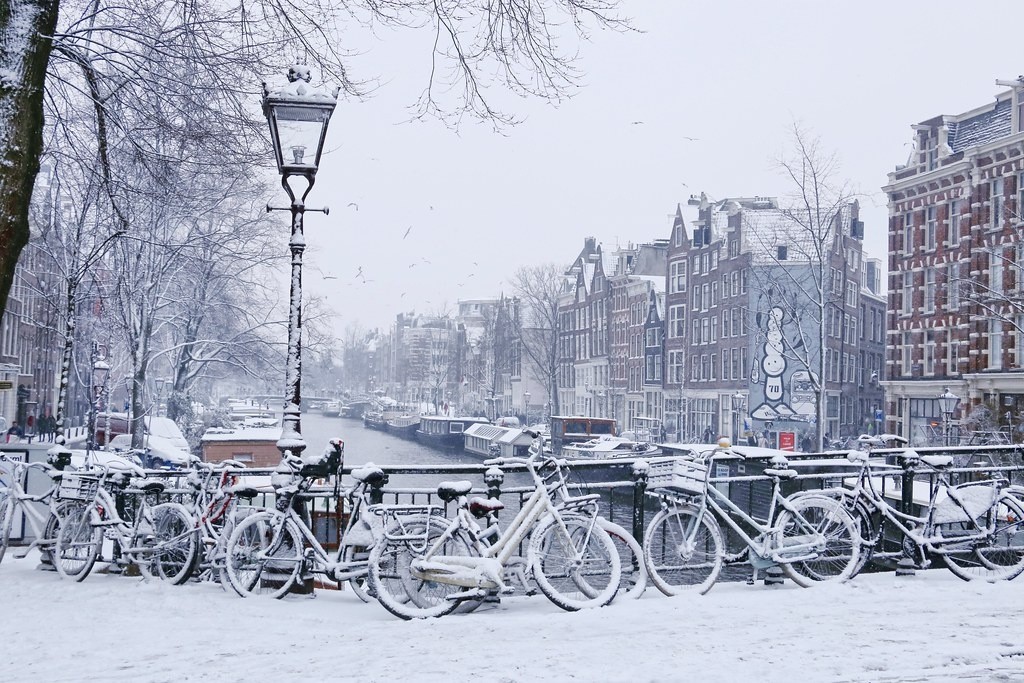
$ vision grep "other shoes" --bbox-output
[42,441,44,442]
[48,440,50,442]
[51,441,52,443]
[38,441,41,442]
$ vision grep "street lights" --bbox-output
[524,390,532,427]
[88,362,112,453]
[259,63,342,596]
[598,391,606,418]
[937,387,959,447]
[732,390,746,447]
[154,375,165,417]
[125,371,134,435]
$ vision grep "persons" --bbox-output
[7,413,56,443]
[112,406,119,412]
[659,426,830,453]
[414,394,526,425]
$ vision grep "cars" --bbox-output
[107,435,201,476]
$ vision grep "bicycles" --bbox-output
[225,437,427,606]
[643,438,863,599]
[402,457,651,617]
[968,485,1024,569]
[791,432,1023,582]
[364,428,620,621]
[0,448,271,595]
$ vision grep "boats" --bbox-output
[319,401,340,415]
[361,407,663,463]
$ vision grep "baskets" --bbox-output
[59,473,117,503]
[645,458,708,497]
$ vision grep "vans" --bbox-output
[95,413,191,457]
